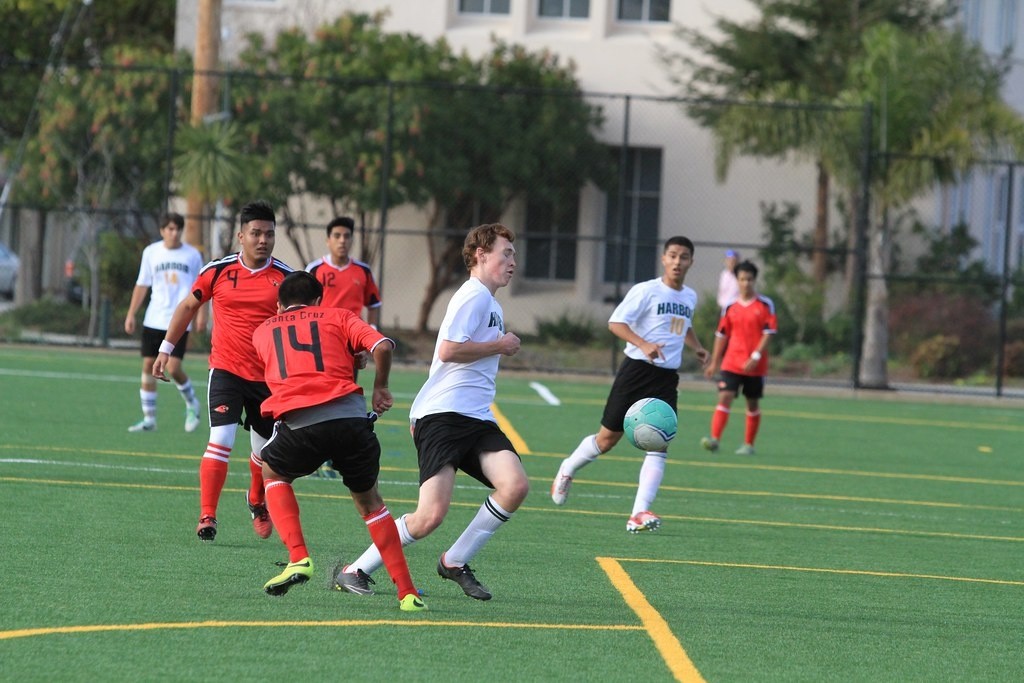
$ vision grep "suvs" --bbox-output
[65,232,82,303]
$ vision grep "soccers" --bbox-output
[623,398,678,452]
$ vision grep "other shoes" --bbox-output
[735,444,756,455]
[321,462,337,479]
[128,419,158,433]
[700,437,719,452]
[184,398,200,433]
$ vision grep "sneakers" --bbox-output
[400,593,429,611]
[245,490,273,539]
[437,553,492,600]
[336,565,376,597]
[551,458,573,505]
[263,557,314,596]
[626,510,662,534]
[196,514,217,541]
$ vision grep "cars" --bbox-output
[0,240,19,301]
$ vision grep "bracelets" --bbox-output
[751,351,761,361]
[158,339,175,355]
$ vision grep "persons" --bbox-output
[152,202,368,541]
[335,224,529,601]
[124,212,210,432]
[700,260,778,455]
[252,270,428,611]
[551,235,710,534]
[305,216,384,332]
[716,249,741,308]
